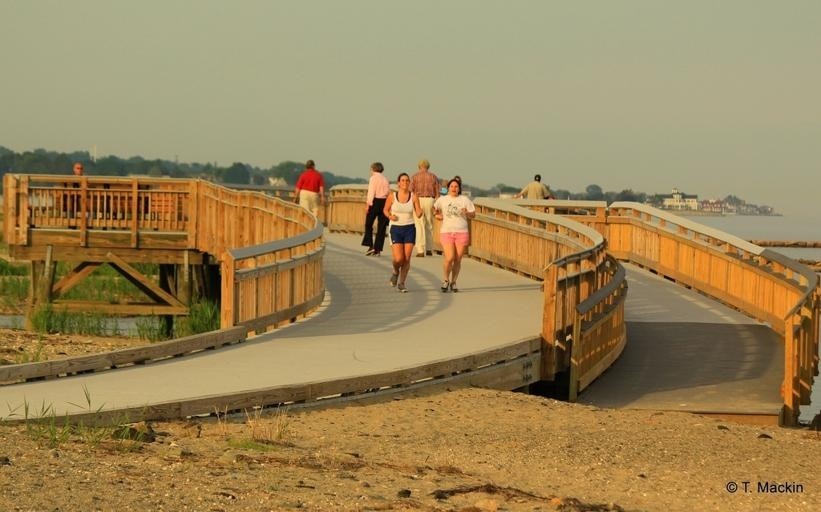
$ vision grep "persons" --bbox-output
[292,159,327,219]
[410,159,441,258]
[65,163,85,231]
[512,173,554,200]
[360,162,391,256]
[432,180,477,294]
[383,172,426,292]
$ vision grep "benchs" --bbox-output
[53,182,153,228]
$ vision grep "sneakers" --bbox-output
[450,282,458,292]
[440,280,450,292]
[426,250,433,256]
[375,251,381,257]
[389,274,399,287]
[397,283,409,293]
[415,252,424,257]
[364,247,376,256]
